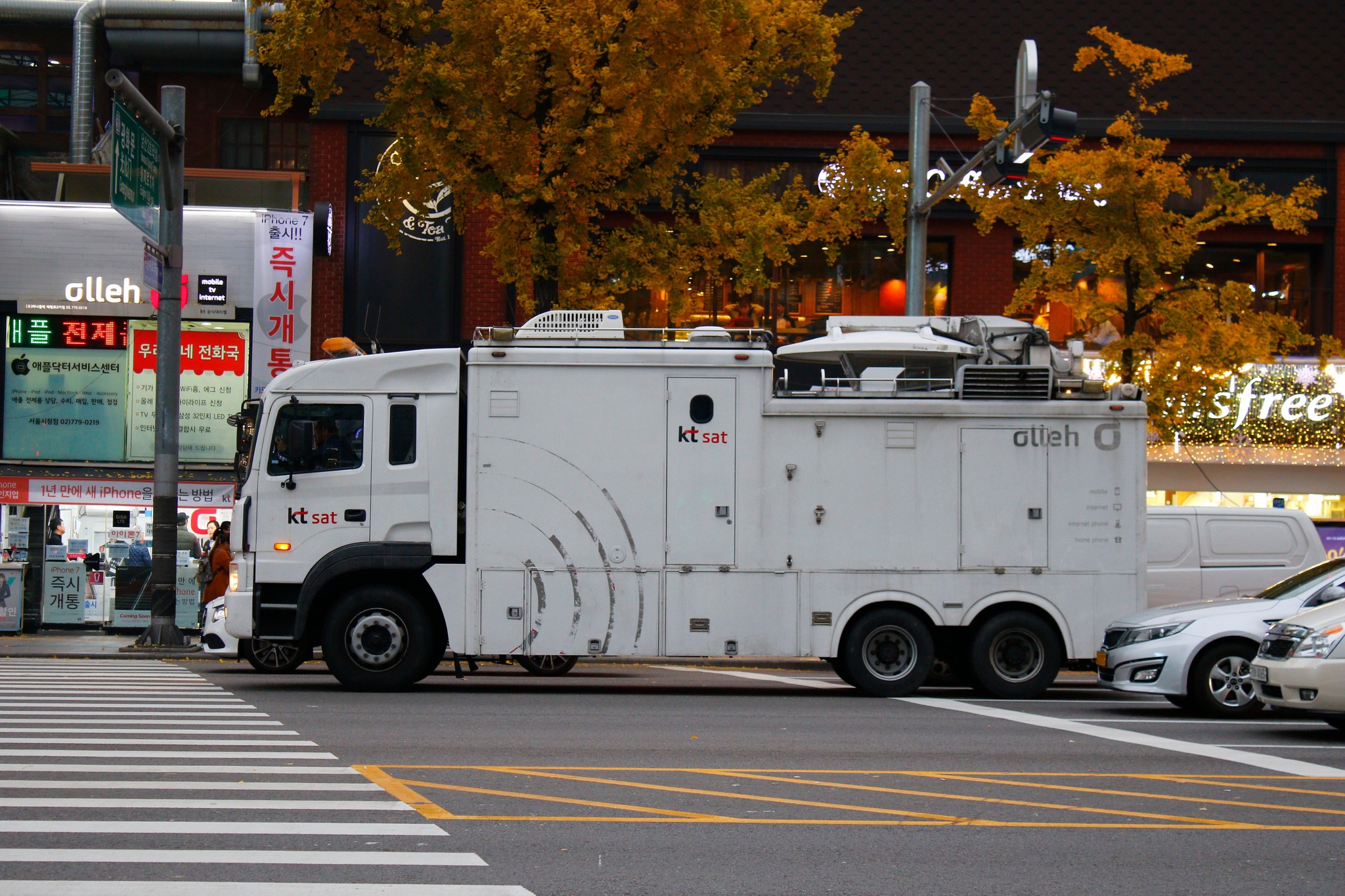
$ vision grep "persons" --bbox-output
[48,519,72,562]
[276,413,359,470]
[203,521,221,556]
[213,521,231,541]
[177,513,203,559]
[125,534,152,567]
[202,533,233,603]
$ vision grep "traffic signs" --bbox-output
[110,98,167,293]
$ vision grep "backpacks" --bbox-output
[196,549,227,583]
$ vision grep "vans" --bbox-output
[1144,505,1329,626]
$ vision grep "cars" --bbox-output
[200,595,595,675]
[1243,591,1345,730]
[1093,552,1345,719]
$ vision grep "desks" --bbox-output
[683,304,834,347]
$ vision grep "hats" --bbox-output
[177,512,190,523]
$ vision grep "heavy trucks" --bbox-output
[230,307,1151,697]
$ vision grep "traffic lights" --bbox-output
[980,149,1027,191]
[1022,107,1079,154]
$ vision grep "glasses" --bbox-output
[141,532,144,535]
